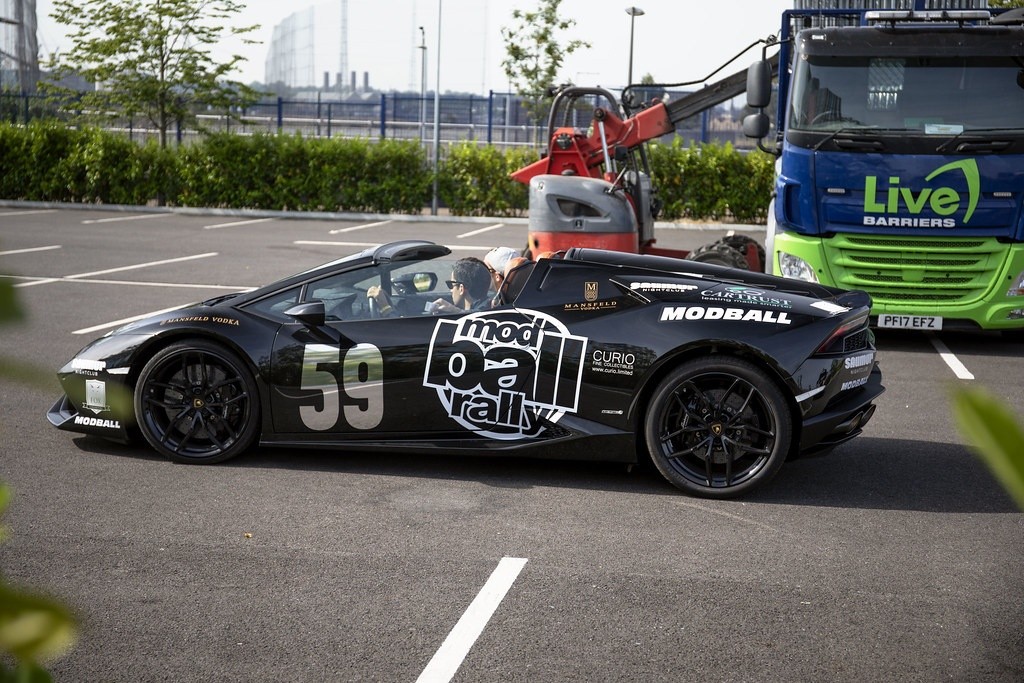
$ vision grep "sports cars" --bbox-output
[47,240,886,499]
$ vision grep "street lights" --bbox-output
[625,6,645,84]
[418,27,427,122]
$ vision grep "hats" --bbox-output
[485,247,520,276]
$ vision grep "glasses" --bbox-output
[445,280,466,289]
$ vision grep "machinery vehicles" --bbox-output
[511,30,780,272]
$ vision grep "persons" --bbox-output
[484,246,521,293]
[367,257,491,314]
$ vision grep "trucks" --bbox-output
[743,8,1024,333]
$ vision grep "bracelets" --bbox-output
[380,305,391,312]
